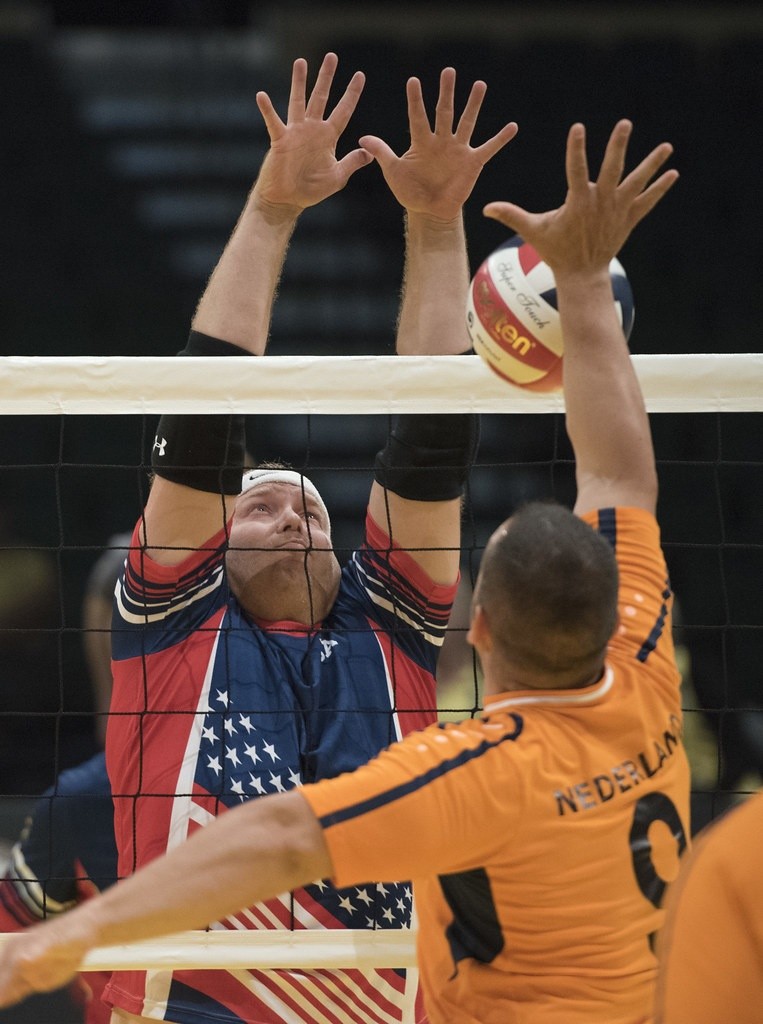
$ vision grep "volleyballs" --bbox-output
[462,229,637,395]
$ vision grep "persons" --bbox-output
[104,53,517,1024]
[0,532,145,932]
[0,120,691,1024]
[658,790,762,1024]
